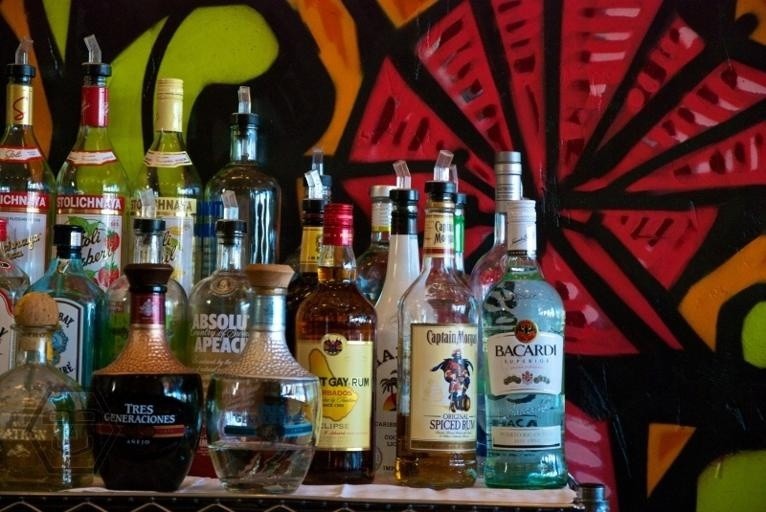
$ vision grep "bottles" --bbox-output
[0,37,51,286]
[51,32,131,275]
[122,78,202,284]
[0,147,571,496]
[202,85,282,265]
